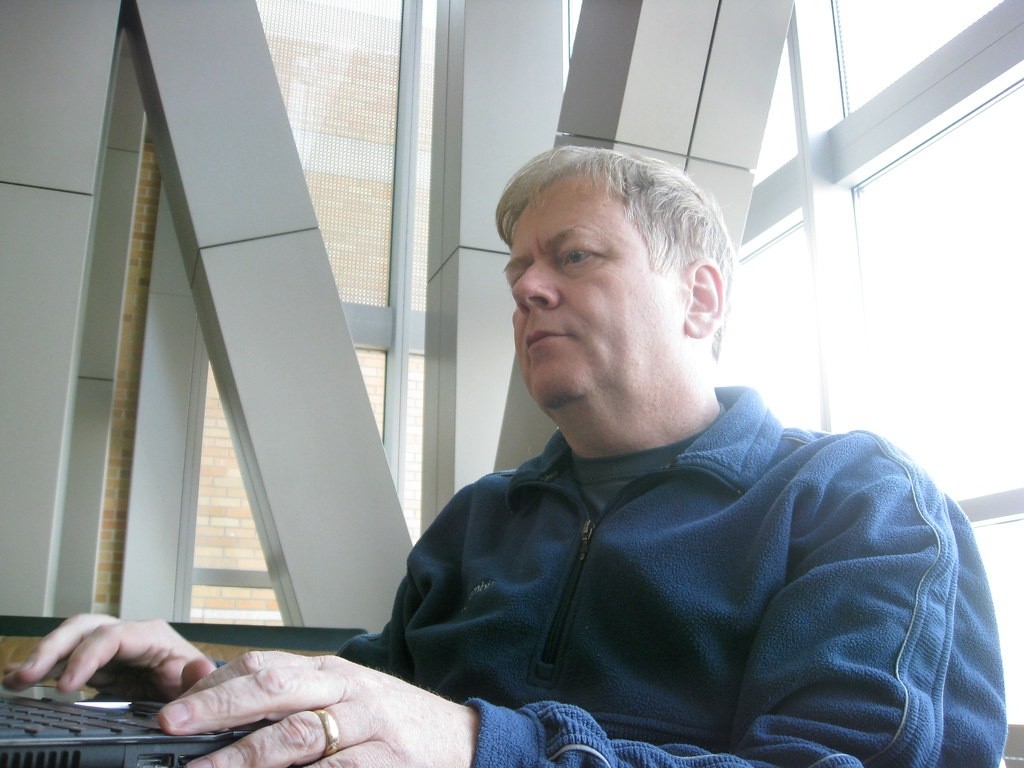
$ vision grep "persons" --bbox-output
[0,142,1011,767]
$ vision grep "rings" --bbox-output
[313,708,340,758]
